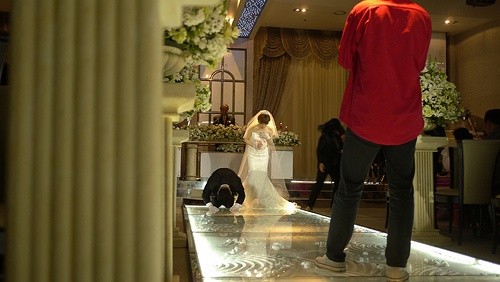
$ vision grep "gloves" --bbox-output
[231,203,243,213]
[251,140,262,150]
[259,131,271,140]
[206,202,219,213]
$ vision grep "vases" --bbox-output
[422,119,437,131]
[164,45,185,76]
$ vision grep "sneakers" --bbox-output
[386,265,410,282]
[306,206,312,212]
[316,254,346,272]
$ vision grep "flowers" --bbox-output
[164,3,240,120]
[190,123,301,153]
[420,53,471,131]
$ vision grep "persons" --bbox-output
[213,104,236,125]
[238,109,298,212]
[442,128,474,172]
[313,0,432,282]
[483,108,500,140]
[305,117,346,211]
[201,167,247,213]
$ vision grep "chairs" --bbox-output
[434,138,500,246]
